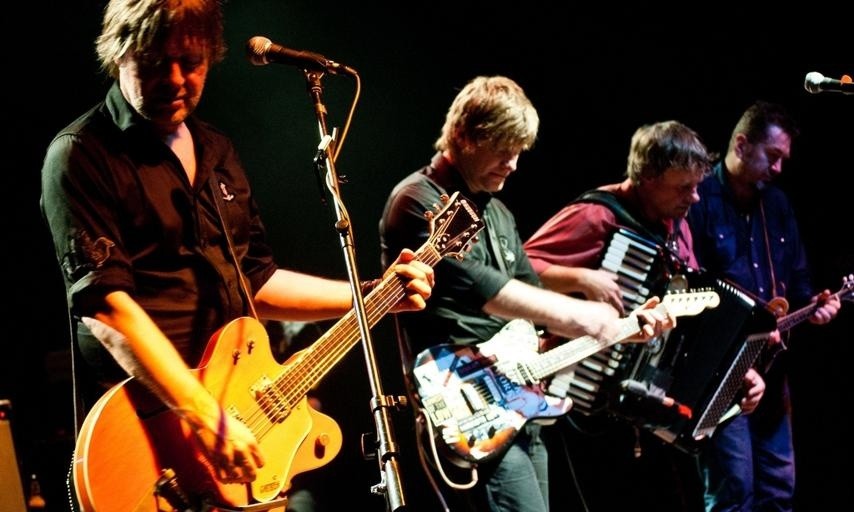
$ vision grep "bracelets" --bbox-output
[359,277,382,299]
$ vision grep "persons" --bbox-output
[40,0,436,511]
[379,76,676,511]
[683,104,841,512]
[522,122,768,511]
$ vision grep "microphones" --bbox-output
[246,35,345,76]
[803,70,854,96]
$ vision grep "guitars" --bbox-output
[412,287,723,471]
[69,192,488,512]
